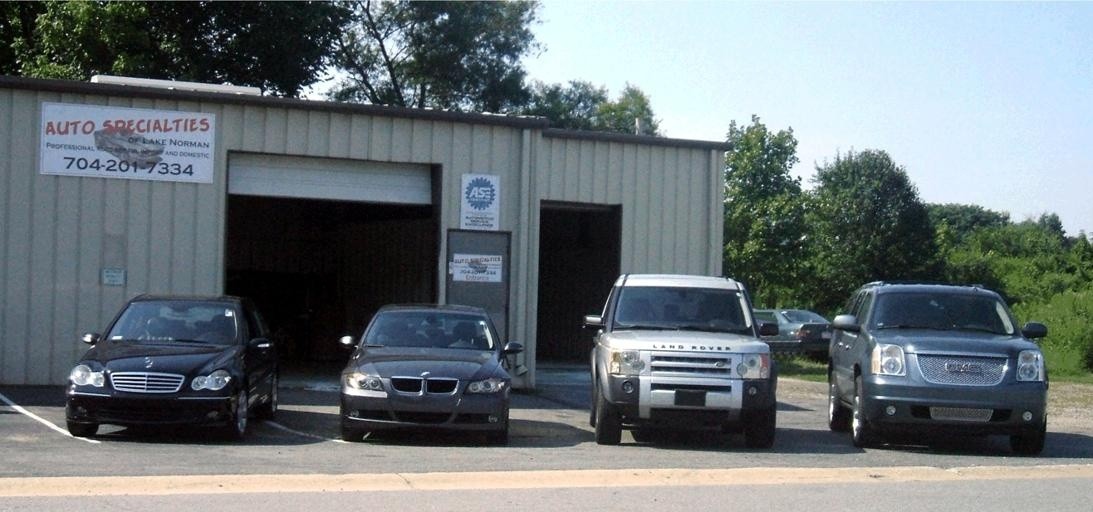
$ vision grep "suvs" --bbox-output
[821,277,1051,457]
[573,272,779,453]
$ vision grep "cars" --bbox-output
[334,301,521,447]
[89,128,166,171]
[751,306,834,359]
[62,290,281,444]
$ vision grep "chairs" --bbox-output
[618,297,656,322]
[195,314,232,343]
[694,294,740,326]
[139,318,178,343]
[441,322,487,350]
